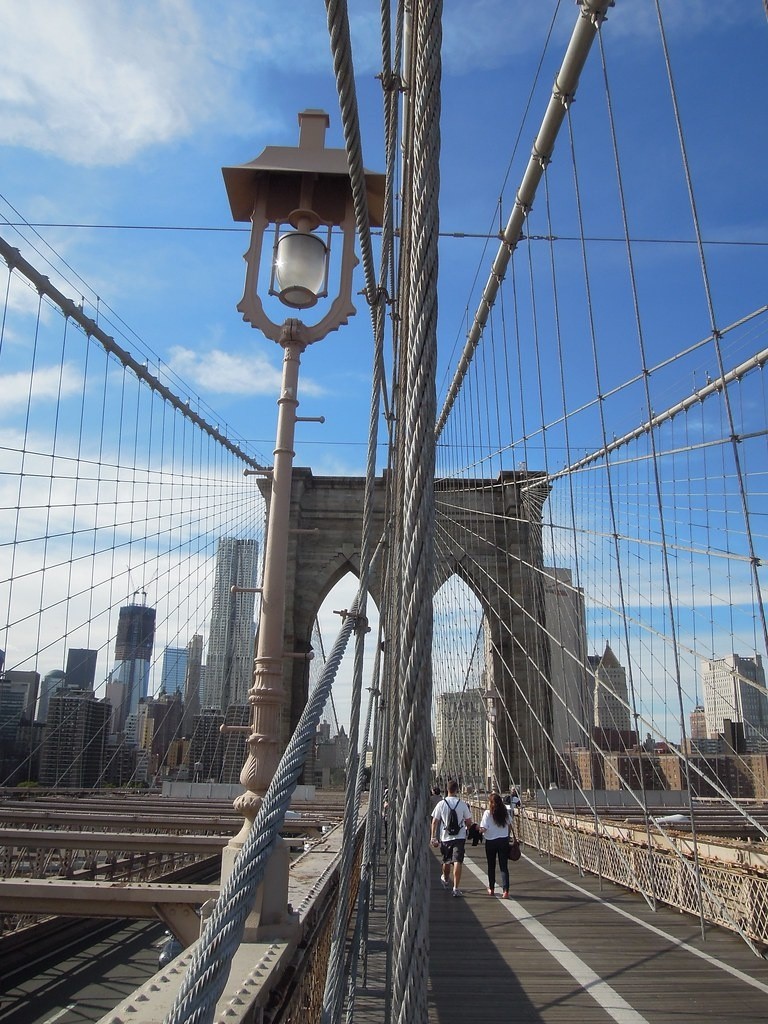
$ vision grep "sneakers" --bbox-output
[440,874,451,889]
[453,887,463,896]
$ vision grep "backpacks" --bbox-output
[440,798,463,835]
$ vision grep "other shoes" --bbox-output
[502,888,509,899]
[487,888,494,896]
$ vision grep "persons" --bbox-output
[511,786,520,806]
[430,787,442,814]
[478,793,514,899]
[431,781,472,897]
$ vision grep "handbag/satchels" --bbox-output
[508,823,521,861]
